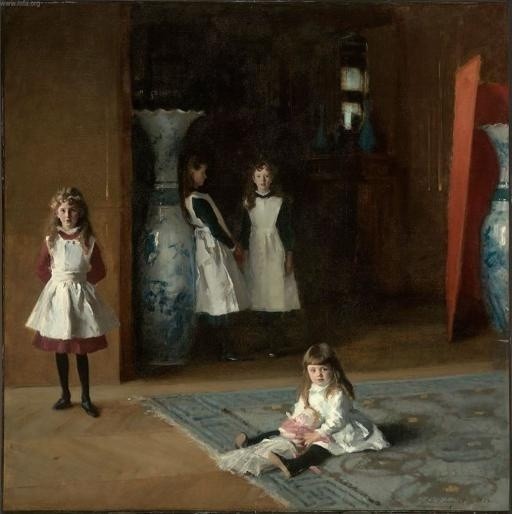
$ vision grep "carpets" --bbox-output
[126,369,510,511]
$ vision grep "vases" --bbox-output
[127,104,210,369]
[480,120,511,343]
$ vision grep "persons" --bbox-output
[25,183,119,418]
[232,155,300,363]
[235,342,362,480]
[176,144,245,365]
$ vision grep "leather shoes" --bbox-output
[234,432,248,448]
[82,400,99,417]
[272,451,296,477]
[53,399,71,408]
